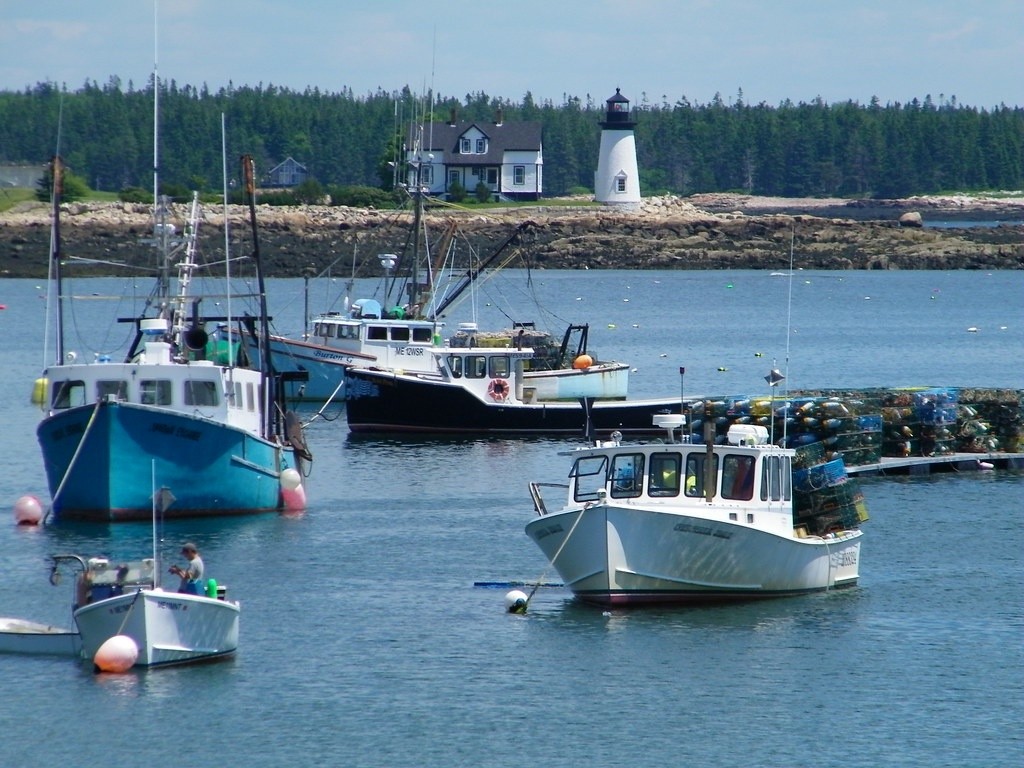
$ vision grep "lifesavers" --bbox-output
[488,379,510,400]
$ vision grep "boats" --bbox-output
[0,614,81,658]
[524,219,868,612]
[37,0,312,520]
[72,457,241,671]
[342,244,701,436]
[214,22,628,404]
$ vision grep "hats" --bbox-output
[180,543,196,553]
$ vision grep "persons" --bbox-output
[169,543,205,593]
